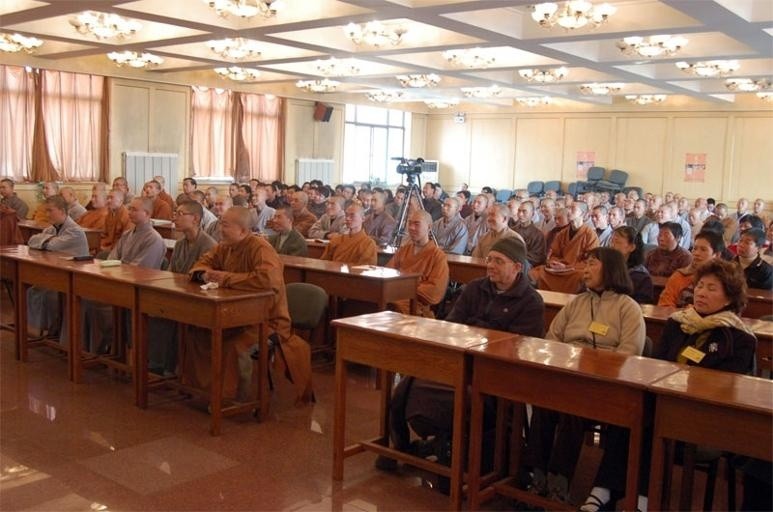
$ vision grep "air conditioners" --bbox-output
[418,158,438,187]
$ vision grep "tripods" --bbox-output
[389,182,440,248]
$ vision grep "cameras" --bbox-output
[393,158,425,184]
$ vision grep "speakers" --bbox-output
[314,101,334,122]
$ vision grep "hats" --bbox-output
[489,236,525,263]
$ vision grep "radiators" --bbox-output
[120,150,178,205]
[294,157,335,189]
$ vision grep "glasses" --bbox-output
[486,256,514,265]
[173,210,195,216]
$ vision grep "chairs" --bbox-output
[493,166,646,200]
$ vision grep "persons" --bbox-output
[1,174,394,412]
[375,183,773,511]
